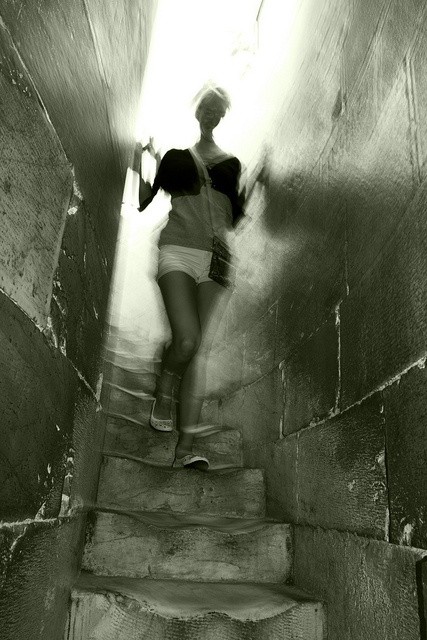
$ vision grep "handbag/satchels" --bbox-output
[208,235,232,288]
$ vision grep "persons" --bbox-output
[133,86,270,470]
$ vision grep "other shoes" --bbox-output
[148,400,173,432]
[172,454,210,471]
